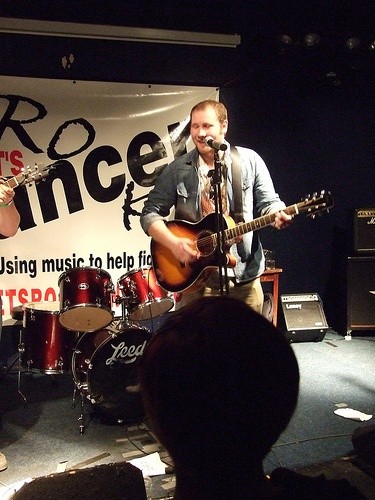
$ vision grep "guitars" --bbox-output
[150,189,335,293]
[0,161,50,190]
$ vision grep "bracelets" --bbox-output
[0,200,13,207]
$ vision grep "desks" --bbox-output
[260,268,282,327]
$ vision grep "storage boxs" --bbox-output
[338,252,375,335]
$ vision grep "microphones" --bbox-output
[204,136,227,151]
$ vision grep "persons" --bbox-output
[0,175,21,472]
[139,296,365,500]
[140,100,294,316]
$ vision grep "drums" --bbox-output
[117,267,175,322]
[19,301,74,374]
[58,266,114,332]
[71,320,153,416]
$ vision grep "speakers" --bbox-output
[258,274,329,343]
[13,462,148,500]
[334,207,375,335]
